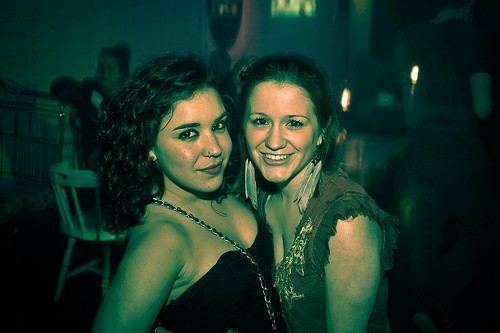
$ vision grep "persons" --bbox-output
[87,53,282,333]
[232,51,401,333]
[51,42,135,174]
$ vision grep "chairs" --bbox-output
[48,166,131,304]
